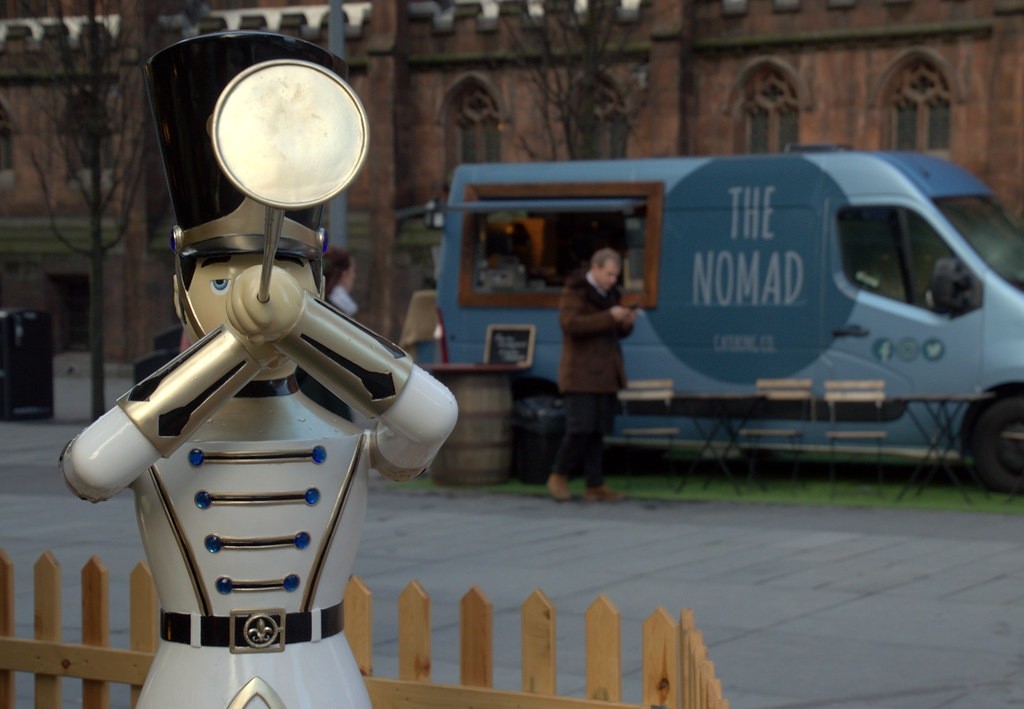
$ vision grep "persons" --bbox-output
[329,260,357,318]
[547,249,636,504]
[61,32,459,709]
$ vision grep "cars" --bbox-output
[0,305,86,423]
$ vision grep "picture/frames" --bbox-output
[483,324,538,368]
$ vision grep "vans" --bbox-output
[430,142,1024,496]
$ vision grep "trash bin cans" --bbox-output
[102,360,136,414]
[49,350,102,424]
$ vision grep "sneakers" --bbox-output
[583,485,624,502]
[547,473,572,501]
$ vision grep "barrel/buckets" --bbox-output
[431,373,513,488]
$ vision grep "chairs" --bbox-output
[826,379,886,500]
[739,377,811,491]
[1002,430,1024,505]
[617,379,686,490]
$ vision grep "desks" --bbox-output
[893,394,996,505]
[674,392,767,496]
